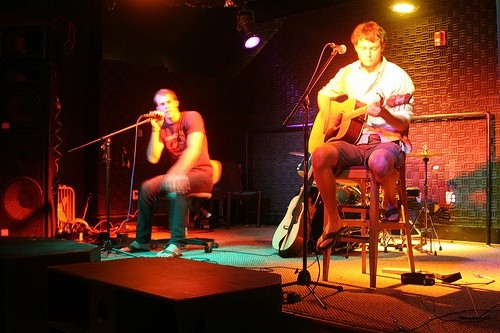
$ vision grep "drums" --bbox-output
[301,186,370,254]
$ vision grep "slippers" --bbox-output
[315,221,350,251]
[157,248,182,257]
[120,245,151,252]
[381,199,401,222]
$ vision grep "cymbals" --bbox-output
[287,150,304,157]
[410,151,444,158]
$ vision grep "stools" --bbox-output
[323,126,414,287]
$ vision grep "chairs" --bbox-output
[153,160,222,252]
[215,161,262,229]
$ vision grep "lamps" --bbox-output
[235,9,261,49]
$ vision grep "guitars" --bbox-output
[306,91,414,155]
[271,172,316,254]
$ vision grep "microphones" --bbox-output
[144,113,165,119]
[330,43,347,55]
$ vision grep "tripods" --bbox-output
[281,50,343,311]
[399,158,443,256]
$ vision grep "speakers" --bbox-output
[0,235,102,333]
[45,256,282,333]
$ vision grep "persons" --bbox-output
[121,89,214,257]
[312,21,414,252]
